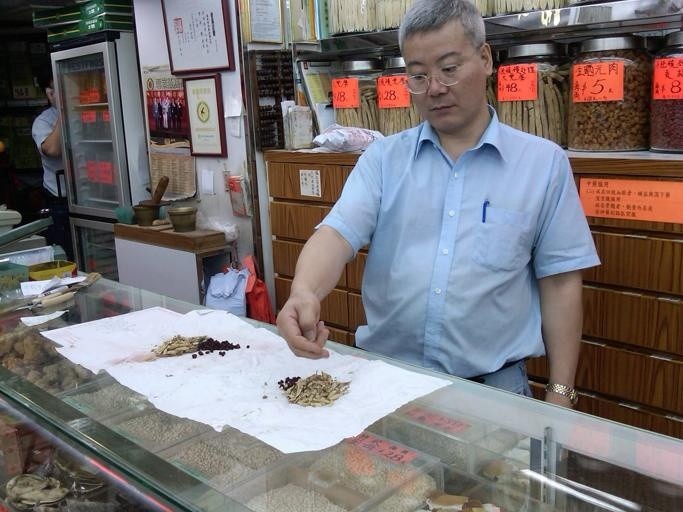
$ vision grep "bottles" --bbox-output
[83,71,109,103]
[167,206,198,233]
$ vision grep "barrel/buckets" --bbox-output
[651,31,680,152]
[379,56,425,135]
[335,60,380,131]
[569,33,650,153]
[500,42,569,151]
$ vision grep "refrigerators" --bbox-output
[48,29,148,283]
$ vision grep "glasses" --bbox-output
[401,47,480,94]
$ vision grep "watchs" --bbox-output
[545,382,579,405]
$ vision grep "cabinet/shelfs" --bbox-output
[262,147,682,435]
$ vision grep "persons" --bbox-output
[274,1,602,410]
[30,75,75,263]
[152,96,183,131]
